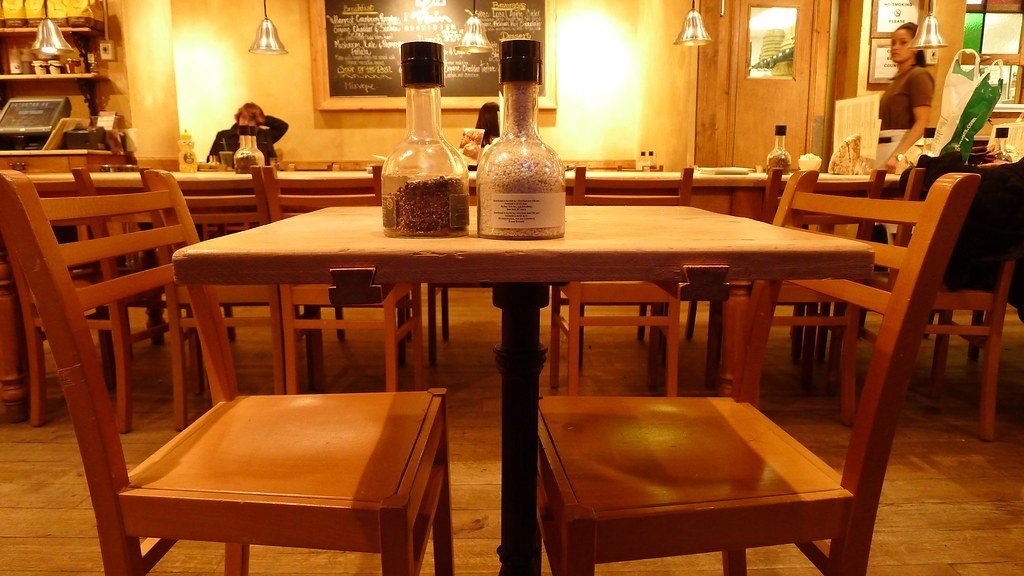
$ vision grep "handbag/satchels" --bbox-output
[931,48,1004,166]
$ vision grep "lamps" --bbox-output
[869,39,898,84]
[907,0,948,50]
[249,0,289,55]
[455,0,495,53]
[673,0,712,46]
[31,0,74,52]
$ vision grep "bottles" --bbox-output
[639,151,655,170]
[270,156,279,179]
[914,127,936,163]
[7,44,34,73]
[987,127,1013,163]
[766,125,791,175]
[380,42,470,239]
[234,135,264,174]
[476,40,565,240]
[177,129,197,173]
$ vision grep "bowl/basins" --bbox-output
[798,159,822,170]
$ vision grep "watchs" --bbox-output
[892,152,903,161]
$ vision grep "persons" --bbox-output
[873,22,935,243]
[207,102,289,166]
[474,102,500,148]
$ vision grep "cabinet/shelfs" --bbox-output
[0,27,103,80]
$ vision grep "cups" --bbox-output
[31,61,60,74]
[219,152,234,172]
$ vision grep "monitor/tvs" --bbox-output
[0,96,72,141]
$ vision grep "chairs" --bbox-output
[1,143,1024,576]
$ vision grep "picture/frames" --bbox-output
[870,0,925,39]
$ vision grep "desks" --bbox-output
[1,160,1023,403]
[170,207,876,576]
